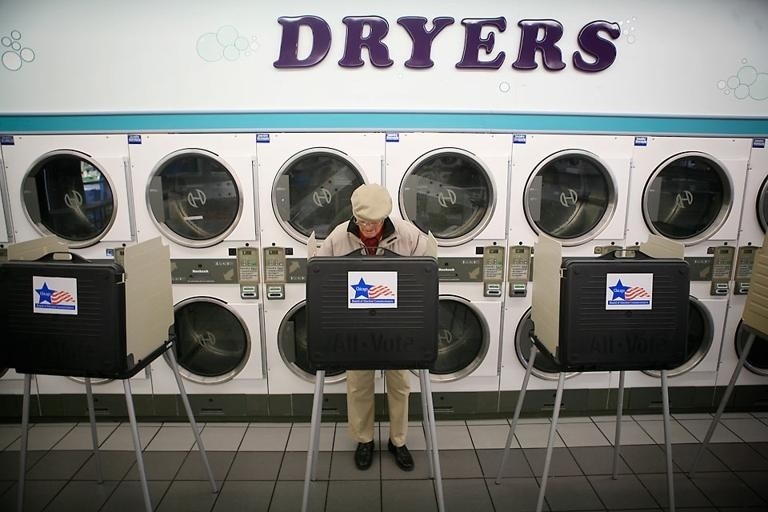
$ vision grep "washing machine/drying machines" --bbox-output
[0,131,768,423]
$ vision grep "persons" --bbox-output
[314,182,438,472]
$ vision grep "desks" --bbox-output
[302,363,444,512]
[14,341,218,512]
[496,330,676,512]
[701,323,767,449]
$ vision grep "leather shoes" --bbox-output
[387,438,413,472]
[354,440,374,470]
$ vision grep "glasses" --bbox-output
[353,218,383,230]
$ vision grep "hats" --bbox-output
[350,183,392,222]
